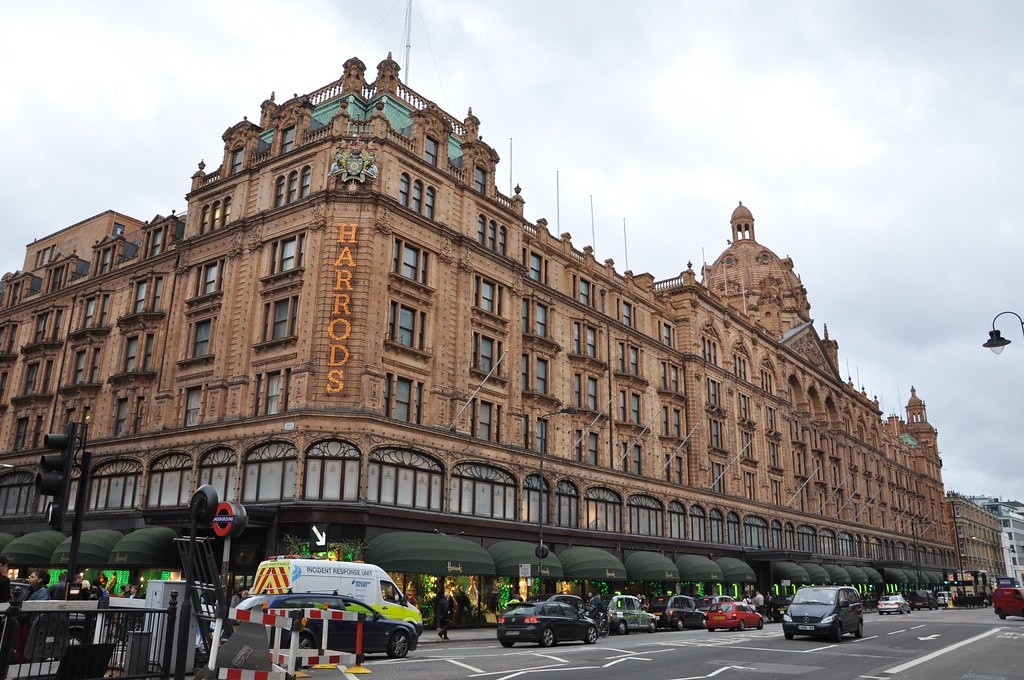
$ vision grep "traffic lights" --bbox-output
[943,572,948,585]
[953,572,958,585]
[869,578,873,588]
[35,422,75,531]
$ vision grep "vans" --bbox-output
[994,588,1024,619]
[254,554,423,658]
[782,586,864,642]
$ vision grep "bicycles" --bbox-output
[587,613,611,638]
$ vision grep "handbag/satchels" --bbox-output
[587,605,595,611]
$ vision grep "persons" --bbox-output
[0,565,246,644]
[862,587,962,611]
[739,592,773,623]
[409,586,650,642]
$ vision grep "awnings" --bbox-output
[366,532,948,583]
[0,528,182,570]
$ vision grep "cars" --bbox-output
[773,594,795,623]
[878,595,911,615]
[696,595,736,613]
[646,595,707,631]
[705,602,763,632]
[53,612,87,661]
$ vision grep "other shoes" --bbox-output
[444,637,449,639]
[438,633,443,639]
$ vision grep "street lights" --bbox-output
[911,521,932,591]
[537,407,578,601]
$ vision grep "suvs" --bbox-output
[588,594,658,635]
[525,593,587,615]
[497,601,598,648]
[233,587,418,659]
[936,591,952,606]
[908,590,938,611]
